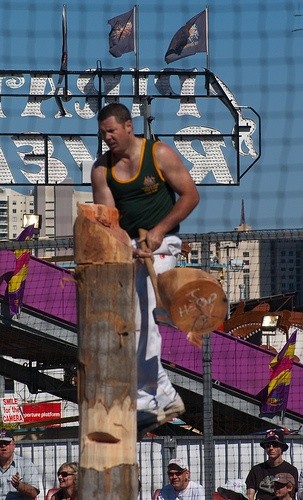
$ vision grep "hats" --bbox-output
[167,459,188,470]
[217,479,250,500]
[270,473,295,485]
[260,429,288,451]
[0,429,13,441]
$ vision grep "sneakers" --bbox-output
[137,403,166,430]
[163,391,186,417]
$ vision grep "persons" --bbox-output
[217,479,249,500]
[89,104,198,430]
[272,472,295,500]
[46,462,79,499]
[154,458,206,500]
[246,428,298,500]
[0,430,40,500]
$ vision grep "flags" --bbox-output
[165,8,208,64]
[107,7,137,59]
[7,225,39,317]
[257,329,297,417]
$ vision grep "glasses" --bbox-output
[264,443,283,450]
[168,470,186,476]
[58,472,74,477]
